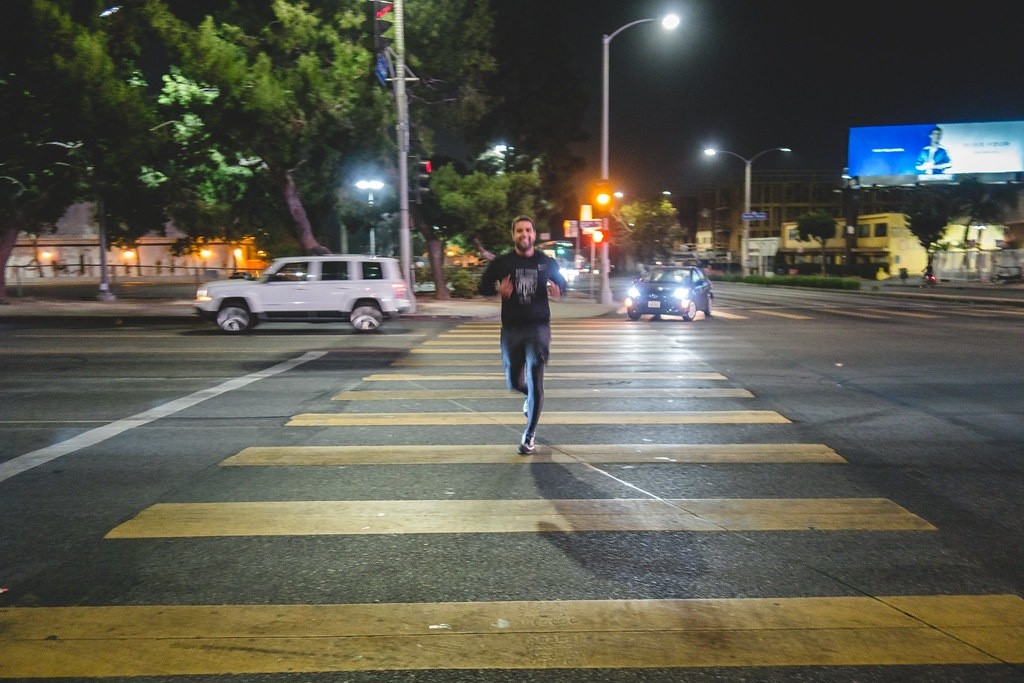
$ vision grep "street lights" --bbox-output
[355,179,384,256]
[704,147,793,279]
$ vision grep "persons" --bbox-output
[478,215,567,455]
[915,127,952,174]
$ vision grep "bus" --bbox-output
[772,247,891,280]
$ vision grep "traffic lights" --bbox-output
[596,179,612,217]
[412,154,433,205]
[556,245,566,256]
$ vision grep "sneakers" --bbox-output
[523,397,529,417]
[518,430,536,454]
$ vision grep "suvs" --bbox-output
[195,253,417,333]
[390,255,436,293]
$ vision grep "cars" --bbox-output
[625,266,713,322]
